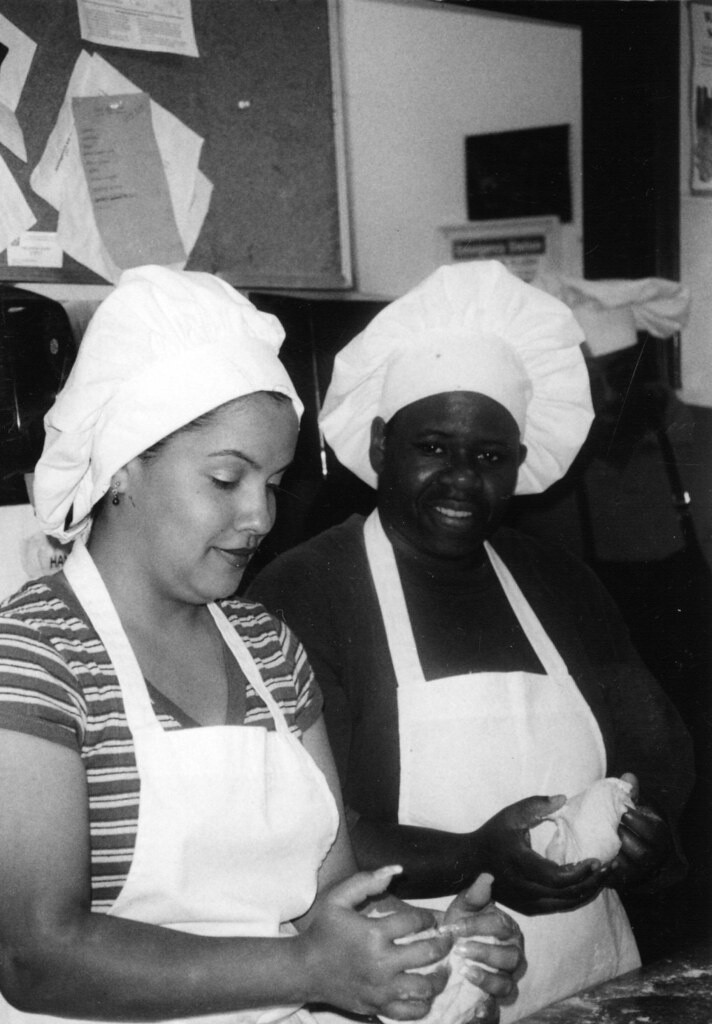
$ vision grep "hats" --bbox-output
[548,275,686,358]
[317,259,595,496]
[35,265,305,544]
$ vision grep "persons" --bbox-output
[244,259,699,1024]
[0,262,525,1024]
